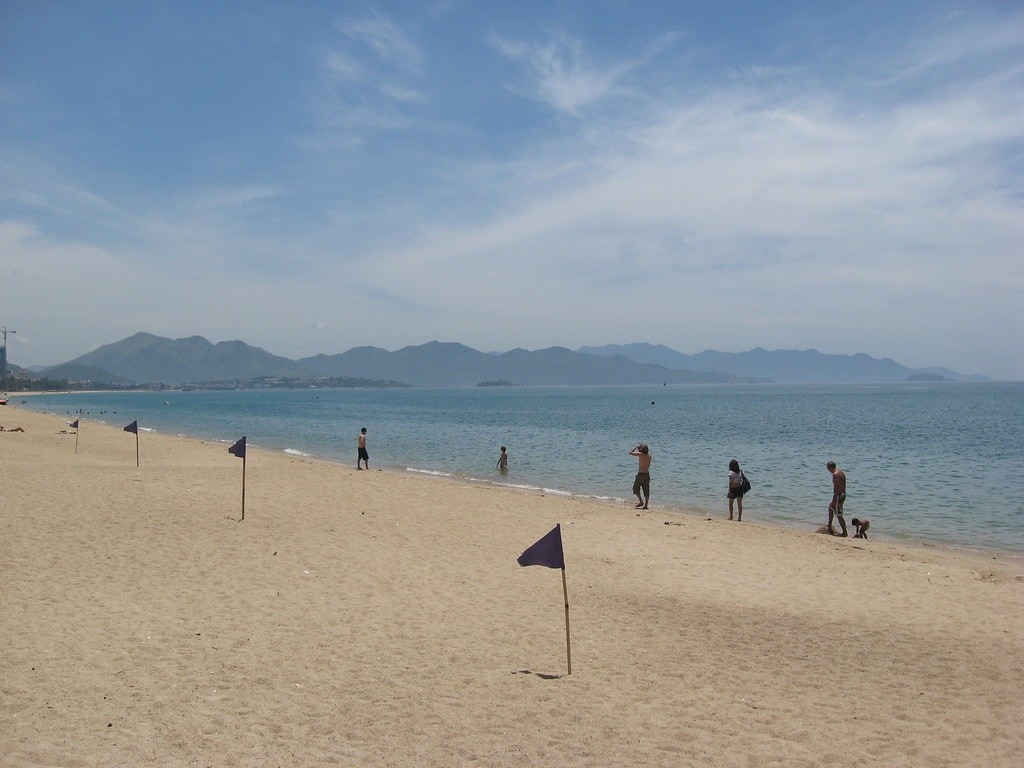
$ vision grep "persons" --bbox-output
[357,427,370,470]
[852,518,870,539]
[728,459,743,521]
[629,443,652,509]
[0,425,25,432]
[826,461,848,537]
[496,446,508,468]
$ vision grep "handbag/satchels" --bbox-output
[739,469,751,494]
